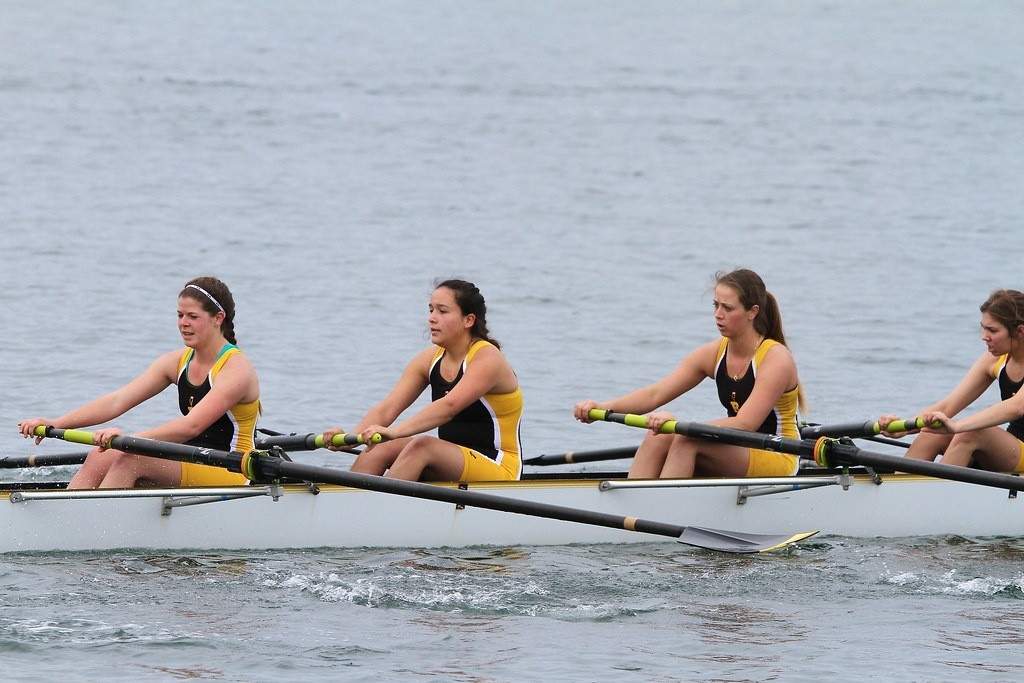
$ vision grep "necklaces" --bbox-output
[728,334,760,382]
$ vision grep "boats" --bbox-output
[0,471,1024,557]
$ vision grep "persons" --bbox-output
[573,269,802,479]
[878,289,1024,476]
[323,279,524,485]
[18,277,260,490]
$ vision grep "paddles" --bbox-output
[585,407,1024,494]
[26,422,824,557]
[521,412,947,468]
[0,432,387,471]
